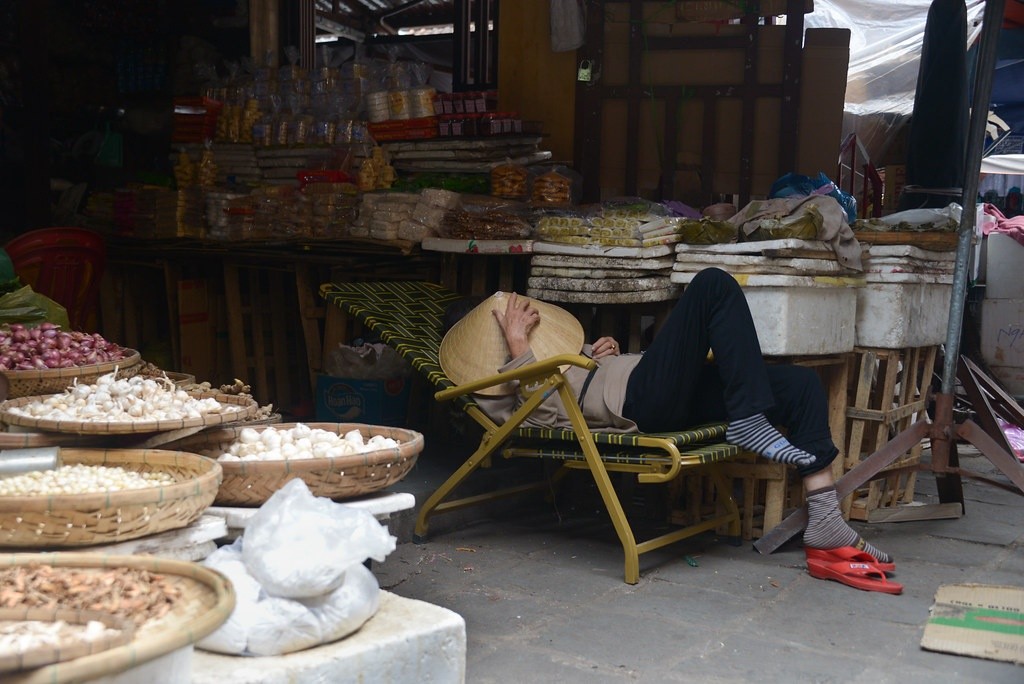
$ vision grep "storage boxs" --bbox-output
[659,239,969,356]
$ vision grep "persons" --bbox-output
[444,266,893,566]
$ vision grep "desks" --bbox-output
[114,232,538,428]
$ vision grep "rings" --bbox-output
[610,343,615,350]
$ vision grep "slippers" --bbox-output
[807,558,905,594]
[805,546,895,571]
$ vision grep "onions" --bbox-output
[0,319,129,370]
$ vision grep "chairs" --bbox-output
[315,280,747,586]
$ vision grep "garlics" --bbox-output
[8,366,400,461]
